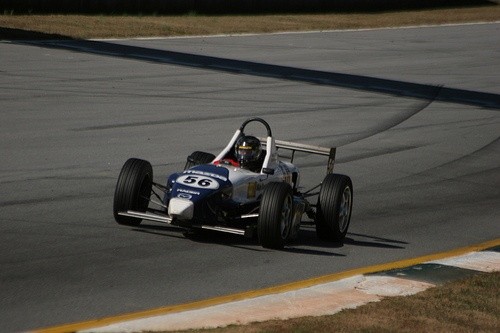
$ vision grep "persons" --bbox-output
[234,135,261,169]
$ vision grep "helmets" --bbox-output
[235,135,262,165]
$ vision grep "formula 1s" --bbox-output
[112,116,353,250]
